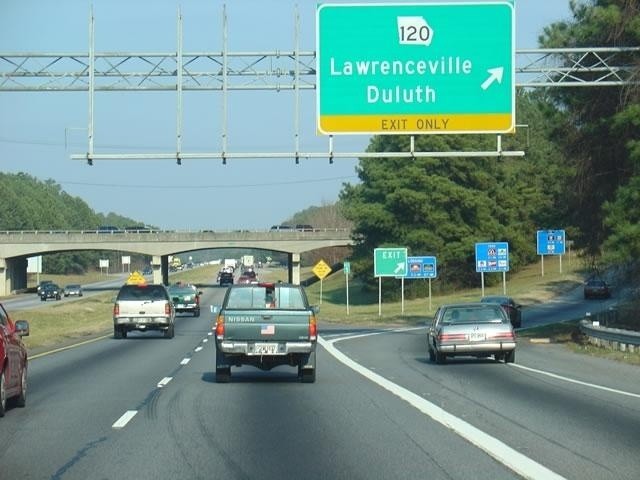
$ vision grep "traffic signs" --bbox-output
[315,0,517,136]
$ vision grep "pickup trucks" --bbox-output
[207,280,322,386]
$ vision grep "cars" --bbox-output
[481,294,524,328]
[295,223,322,232]
[165,281,204,318]
[125,226,159,233]
[86,225,124,234]
[0,298,29,417]
[35,280,84,301]
[424,301,519,365]
[268,225,301,232]
[584,279,610,300]
[216,255,259,286]
[141,262,194,276]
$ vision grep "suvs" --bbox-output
[113,282,178,340]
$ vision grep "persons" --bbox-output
[452,311,459,320]
